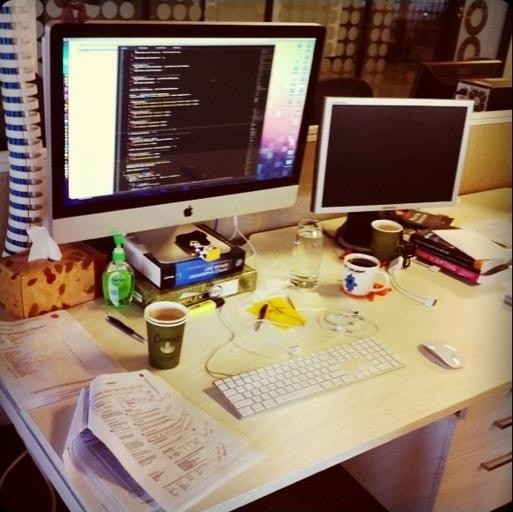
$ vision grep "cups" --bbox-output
[342,253,387,295]
[143,301,189,370]
[144,302,188,325]
[370,220,402,261]
[289,220,322,288]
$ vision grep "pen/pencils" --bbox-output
[107,315,147,341]
[256,304,268,332]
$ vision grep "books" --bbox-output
[409,224,512,284]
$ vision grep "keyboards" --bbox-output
[213,337,405,419]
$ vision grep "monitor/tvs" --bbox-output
[310,97,476,255]
[43,21,327,264]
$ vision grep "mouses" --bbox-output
[422,339,464,369]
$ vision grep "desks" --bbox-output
[0,170,512,512]
